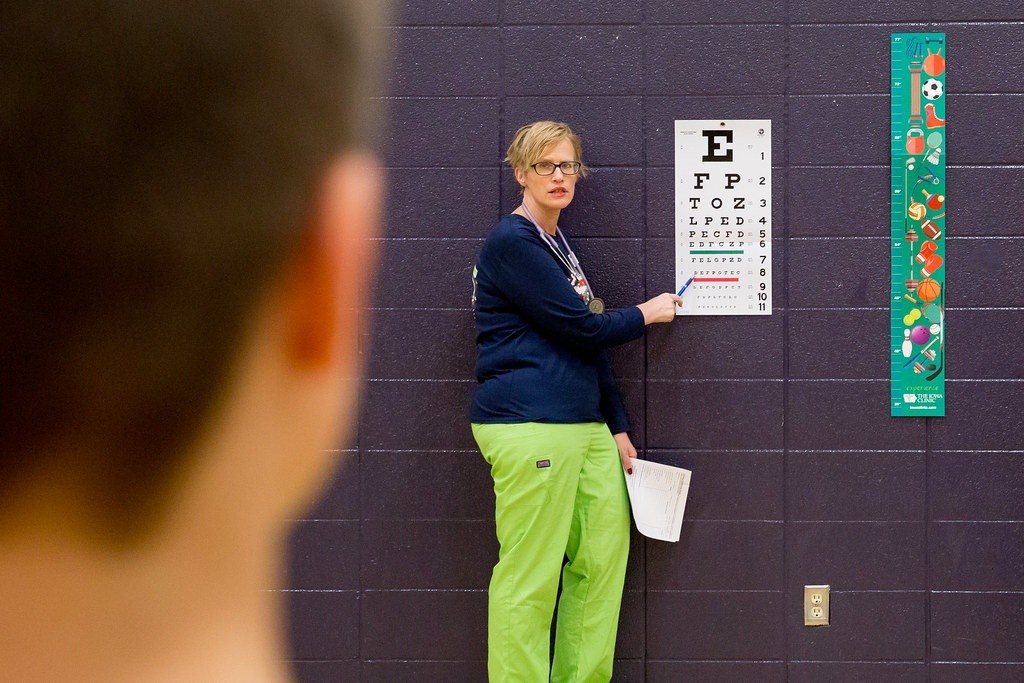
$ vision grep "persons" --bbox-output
[470,121,682,683]
[0,0,385,683]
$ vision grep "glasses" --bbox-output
[530,161,581,176]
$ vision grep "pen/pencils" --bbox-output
[673,275,695,304]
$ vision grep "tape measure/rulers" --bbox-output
[890,33,945,417]
